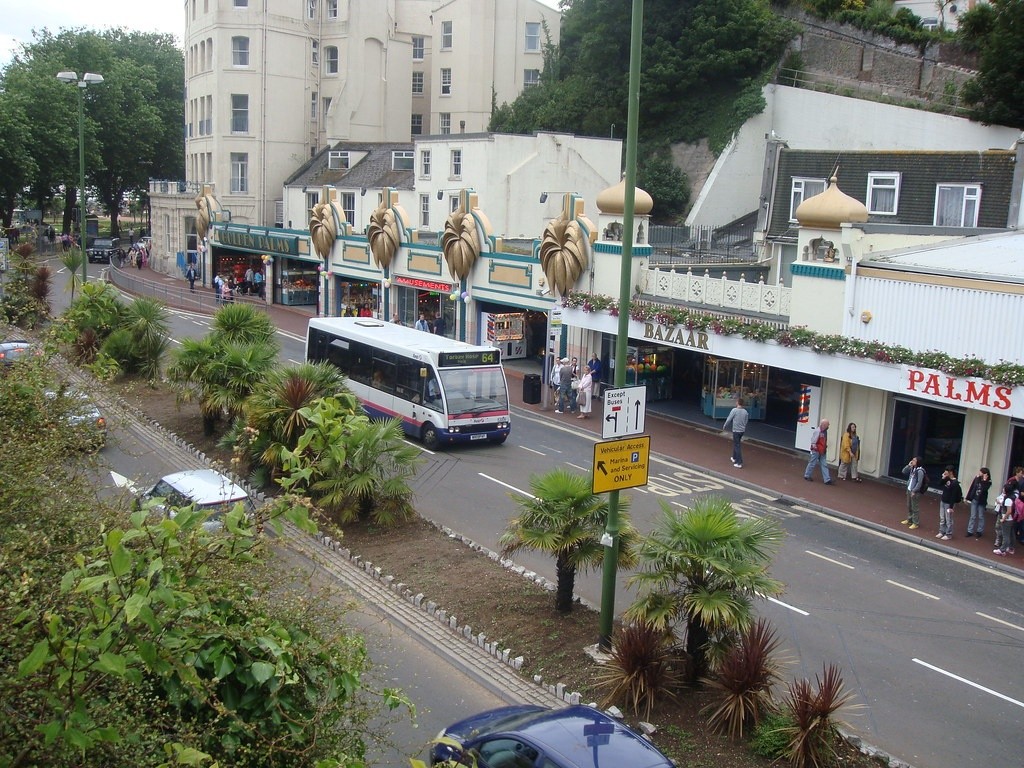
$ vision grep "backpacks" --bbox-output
[915,467,929,493]
[1007,495,1024,521]
[950,480,965,503]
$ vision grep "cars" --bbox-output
[45,392,107,450]
[430,703,676,768]
[0,339,38,373]
[130,236,152,249]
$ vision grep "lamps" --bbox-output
[436,188,474,201]
[540,191,578,203]
[301,185,334,193]
[360,186,394,197]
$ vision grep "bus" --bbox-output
[305,317,510,450]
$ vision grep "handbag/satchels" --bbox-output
[576,390,586,406]
[214,283,219,289]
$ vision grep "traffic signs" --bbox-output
[592,435,651,494]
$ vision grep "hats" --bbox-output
[560,358,569,362]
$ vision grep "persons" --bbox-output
[186,262,198,293]
[140,230,144,238]
[429,373,441,401]
[803,419,836,484]
[723,398,749,468]
[965,468,992,538]
[117,239,151,270]
[389,312,446,336]
[993,467,1024,557]
[901,456,926,530]
[550,353,602,419]
[215,265,264,306]
[129,227,134,242]
[118,220,122,231]
[62,233,81,252]
[838,423,863,482]
[344,298,374,317]
[935,464,962,540]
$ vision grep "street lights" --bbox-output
[57,71,104,294]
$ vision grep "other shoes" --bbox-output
[734,463,742,468]
[731,457,736,462]
[909,523,918,529]
[941,535,951,540]
[804,477,813,481]
[994,538,1001,546]
[841,478,845,481]
[576,414,587,418]
[1005,547,1015,555]
[900,519,912,524]
[572,410,576,413]
[975,532,984,537]
[993,549,1006,556]
[555,409,563,413]
[824,480,832,484]
[935,533,944,539]
[854,478,862,482]
[965,531,973,537]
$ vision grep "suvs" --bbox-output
[87,236,121,263]
[137,468,263,556]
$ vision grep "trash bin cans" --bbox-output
[523,374,541,404]
